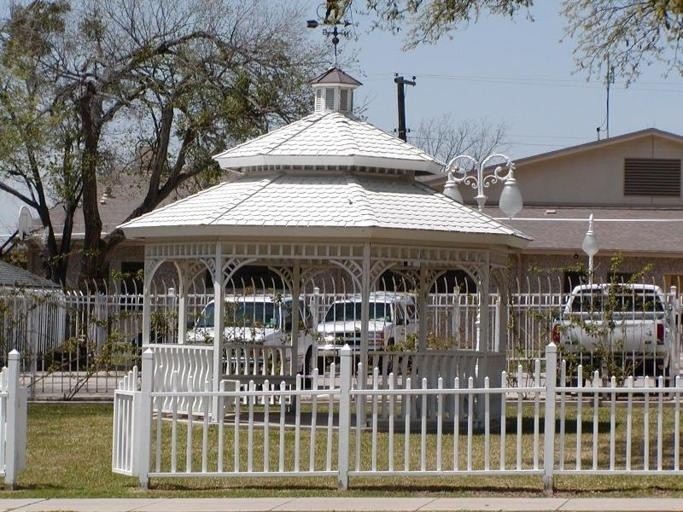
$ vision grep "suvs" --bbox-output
[313,291,419,377]
[186,293,318,390]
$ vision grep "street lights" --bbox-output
[581,210,599,284]
[439,152,525,377]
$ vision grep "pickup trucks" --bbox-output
[549,282,673,395]
